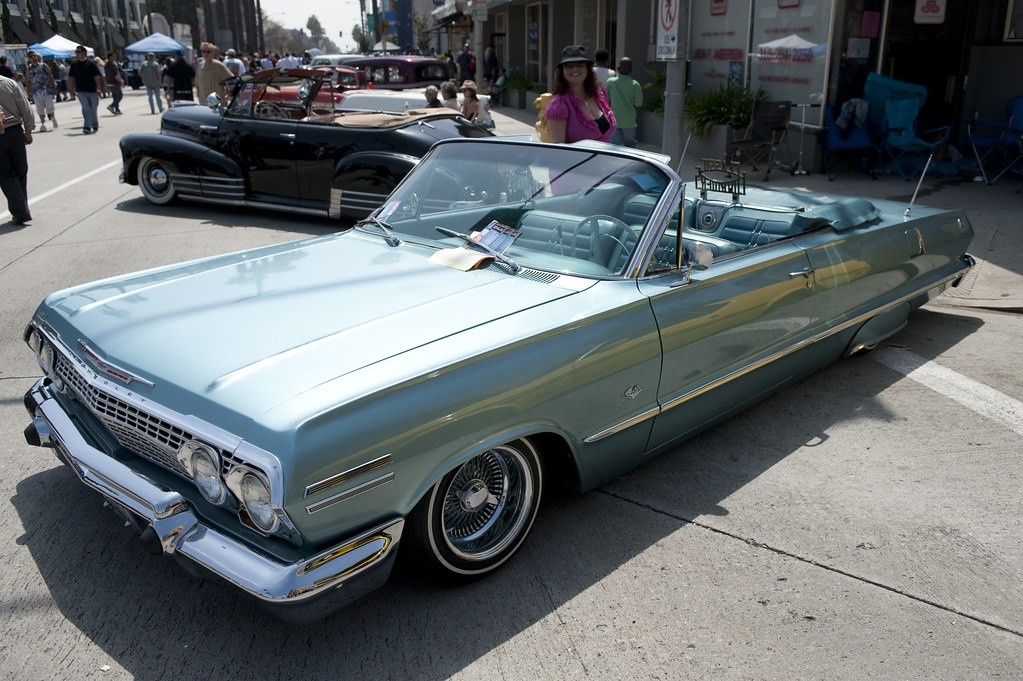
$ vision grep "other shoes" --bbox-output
[93,127,97,131]
[40,125,48,132]
[12,215,32,224]
[107,105,116,114]
[83,127,91,134]
[52,118,58,128]
[116,110,123,114]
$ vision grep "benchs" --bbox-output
[301,110,373,121]
[614,192,802,252]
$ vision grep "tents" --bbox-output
[123,31,190,55]
[803,41,828,55]
[28,34,96,62]
[757,34,819,87]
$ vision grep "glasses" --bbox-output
[75,51,81,53]
[562,62,586,69]
[201,50,211,54]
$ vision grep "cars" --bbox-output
[215,46,491,125]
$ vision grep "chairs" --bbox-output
[727,99,798,182]
[513,209,625,266]
[820,93,1023,185]
[603,224,735,278]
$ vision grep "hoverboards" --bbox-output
[772,103,822,176]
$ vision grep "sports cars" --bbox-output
[117,65,546,227]
[20,135,979,627]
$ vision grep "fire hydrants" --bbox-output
[531,93,554,144]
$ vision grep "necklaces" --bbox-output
[580,97,591,106]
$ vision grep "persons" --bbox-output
[26,51,59,131]
[68,45,103,134]
[439,81,462,111]
[89,51,125,115]
[390,43,507,107]
[545,45,618,196]
[604,56,644,147]
[591,48,615,101]
[160,50,196,110]
[420,84,444,108]
[303,52,312,64]
[138,52,165,114]
[222,47,303,76]
[458,80,479,121]
[0,55,36,222]
[51,58,76,102]
[196,42,236,106]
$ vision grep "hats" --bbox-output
[555,45,594,68]
[225,48,236,56]
[459,79,477,92]
